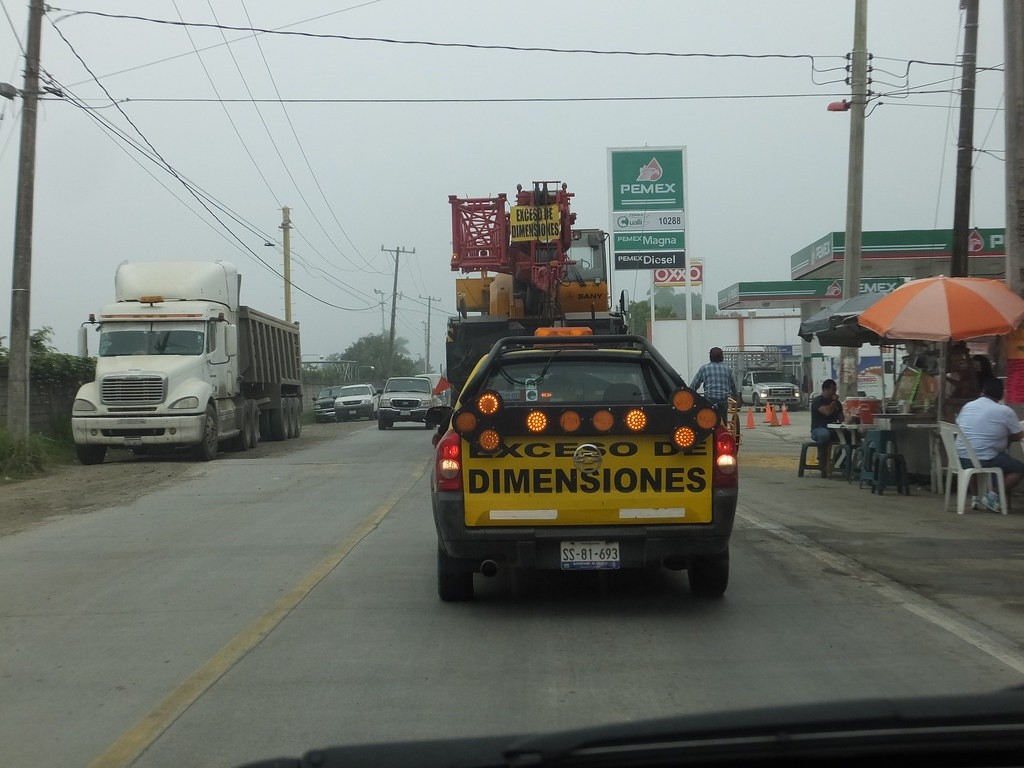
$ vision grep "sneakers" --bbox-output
[981,493,1001,513]
[972,495,986,510]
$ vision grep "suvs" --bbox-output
[333,384,379,422]
[742,368,802,412]
[312,386,343,423]
[377,376,436,431]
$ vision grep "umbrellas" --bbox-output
[858,274,1024,457]
[798,291,926,414]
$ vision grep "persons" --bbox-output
[954,376,1024,513]
[689,347,742,428]
[810,378,861,479]
[792,374,800,390]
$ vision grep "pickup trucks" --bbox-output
[424,325,739,601]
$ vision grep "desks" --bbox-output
[907,424,948,495]
[827,424,877,481]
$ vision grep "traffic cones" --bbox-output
[745,407,756,429]
[762,402,772,423]
[766,405,781,427]
[781,403,791,426]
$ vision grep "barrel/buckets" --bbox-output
[845,396,865,414]
[860,395,883,423]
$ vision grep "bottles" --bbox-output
[844,412,860,424]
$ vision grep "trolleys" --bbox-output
[697,393,743,454]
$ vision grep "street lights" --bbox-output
[0,82,39,479]
[264,242,292,323]
[420,320,427,375]
[374,288,385,333]
[826,93,871,408]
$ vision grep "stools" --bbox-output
[798,442,827,478]
[859,430,898,489]
[871,454,910,496]
[831,444,862,484]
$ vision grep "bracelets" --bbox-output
[831,399,838,402]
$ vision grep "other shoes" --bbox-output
[822,469,827,477]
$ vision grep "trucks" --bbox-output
[415,374,451,408]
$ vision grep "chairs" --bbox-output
[938,421,1007,515]
[603,383,643,403]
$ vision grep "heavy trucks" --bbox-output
[73,259,303,461]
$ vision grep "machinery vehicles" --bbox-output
[443,179,630,409]
